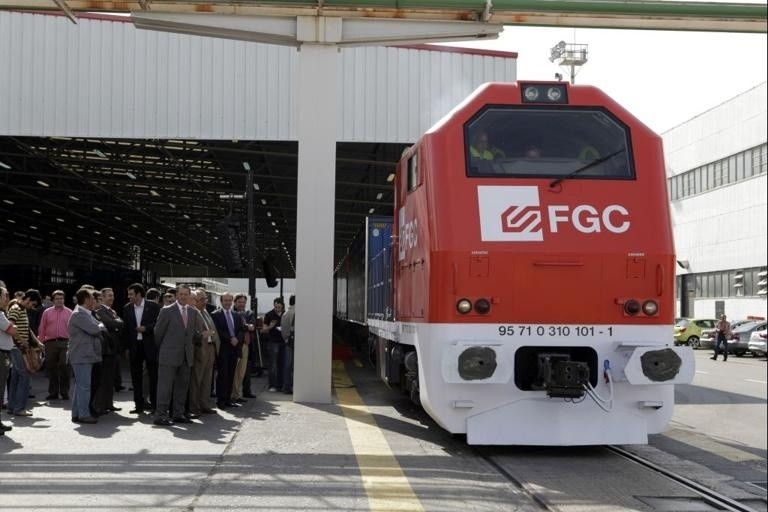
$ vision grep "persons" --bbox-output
[575,136,601,162]
[470,131,505,161]
[710,314,731,361]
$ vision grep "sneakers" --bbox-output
[7,408,14,415]
[60,392,70,400]
[45,393,59,400]
[0,420,13,436]
[14,407,34,416]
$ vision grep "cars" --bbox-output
[674,314,767,356]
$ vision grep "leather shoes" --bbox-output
[71,383,293,427]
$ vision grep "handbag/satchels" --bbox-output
[20,345,47,375]
[286,313,295,350]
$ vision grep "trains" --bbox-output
[333,73,696,445]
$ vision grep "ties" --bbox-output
[240,313,251,345]
[225,310,236,338]
[200,309,218,343]
[181,306,188,328]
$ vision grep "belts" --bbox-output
[207,341,215,345]
